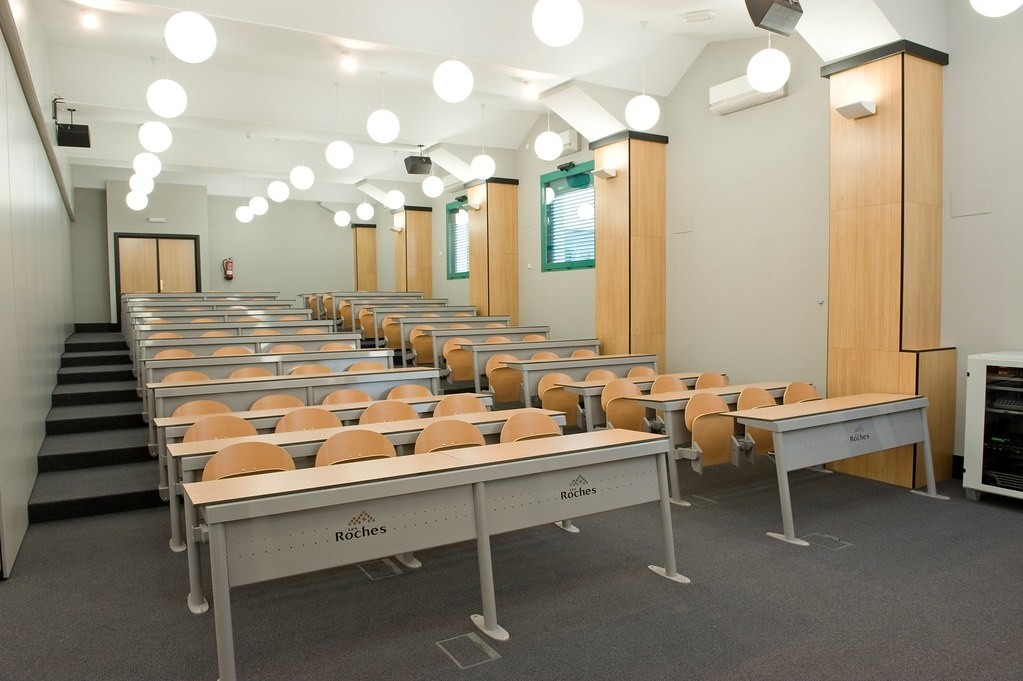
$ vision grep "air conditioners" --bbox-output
[707,70,787,117]
[556,129,581,159]
[440,173,465,193]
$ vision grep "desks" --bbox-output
[122,291,952,681]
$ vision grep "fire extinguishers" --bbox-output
[222,256,233,278]
